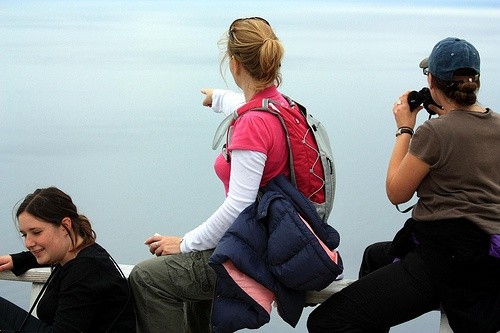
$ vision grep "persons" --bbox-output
[307,37,500,333]
[0,187,138,333]
[128,18,335,333]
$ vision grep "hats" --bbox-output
[420,37,480,81]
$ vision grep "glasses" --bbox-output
[423,67,430,75]
[229,16,267,40]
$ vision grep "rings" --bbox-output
[155,242,160,247]
[397,101,402,105]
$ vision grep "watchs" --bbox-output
[395,128,413,137]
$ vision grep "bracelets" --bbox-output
[398,126,414,131]
